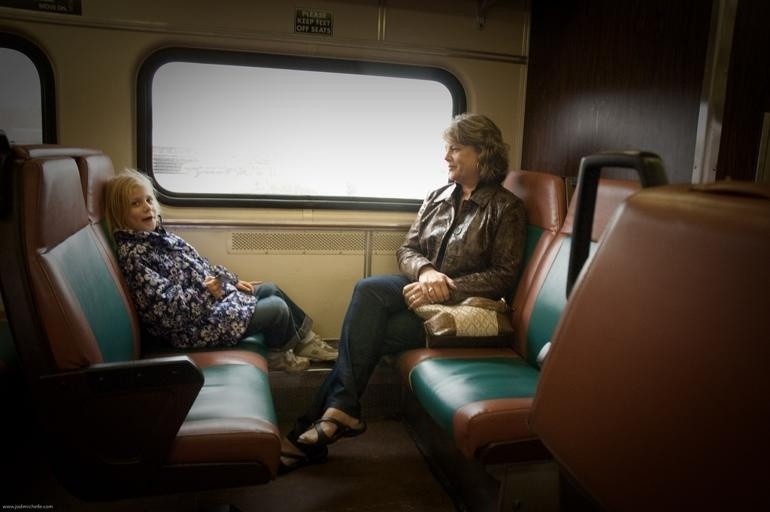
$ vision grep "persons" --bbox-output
[276,113,528,476]
[105,174,339,375]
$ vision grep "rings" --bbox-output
[411,294,418,300]
[428,287,434,294]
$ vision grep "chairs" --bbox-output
[20,145,283,501]
[398,168,770,511]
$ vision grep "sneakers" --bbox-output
[267,349,310,375]
[294,335,340,362]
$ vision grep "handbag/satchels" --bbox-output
[414,292,514,349]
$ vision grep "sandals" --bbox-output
[296,413,366,448]
[275,442,330,475]
